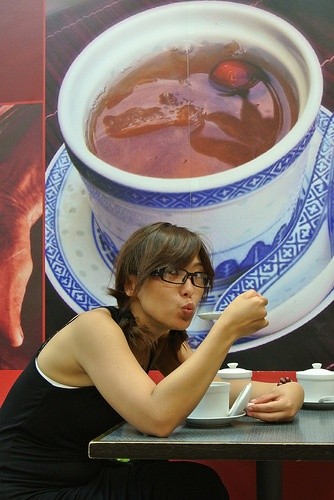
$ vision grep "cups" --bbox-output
[199,382,230,417]
[295,362,334,400]
[216,361,253,406]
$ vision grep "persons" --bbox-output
[0,221,304,500]
[0,113,53,347]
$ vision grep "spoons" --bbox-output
[198,310,223,321]
[212,113,333,335]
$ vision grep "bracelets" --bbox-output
[276,375,298,396]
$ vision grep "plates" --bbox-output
[45,106,333,355]
[188,413,248,428]
[302,398,333,410]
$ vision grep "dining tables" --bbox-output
[88,377,334,499]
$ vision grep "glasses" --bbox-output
[130,267,213,289]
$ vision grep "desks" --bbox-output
[46,1,334,372]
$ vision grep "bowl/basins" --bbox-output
[56,0,325,281]
